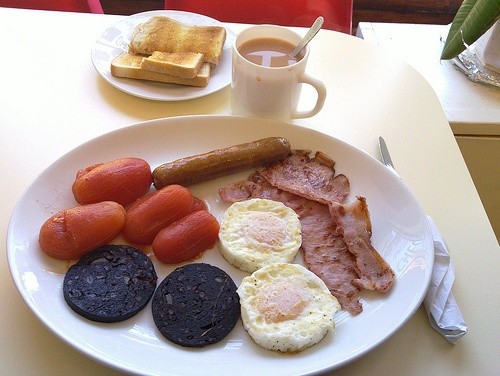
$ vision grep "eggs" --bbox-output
[216,198,303,273]
[236,263,339,353]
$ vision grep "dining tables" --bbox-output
[0,5,500,376]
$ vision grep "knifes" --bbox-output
[379,135,396,171]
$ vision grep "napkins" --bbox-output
[387,162,469,346]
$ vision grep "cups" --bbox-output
[231,25,327,123]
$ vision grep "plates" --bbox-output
[92,10,237,102]
[6,116,436,376]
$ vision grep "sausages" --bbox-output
[152,137,292,188]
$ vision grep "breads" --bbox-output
[110,16,226,88]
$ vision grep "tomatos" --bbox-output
[37,159,220,264]
